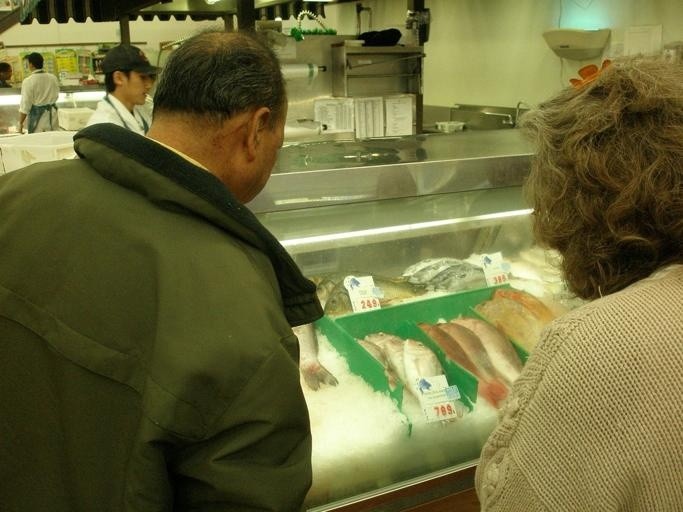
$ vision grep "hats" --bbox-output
[100,44,162,75]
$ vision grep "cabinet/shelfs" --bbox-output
[331,43,426,134]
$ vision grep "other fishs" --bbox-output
[304,255,569,425]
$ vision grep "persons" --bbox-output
[475,56,683,512]
[0,31,325,512]
[0,44,163,137]
